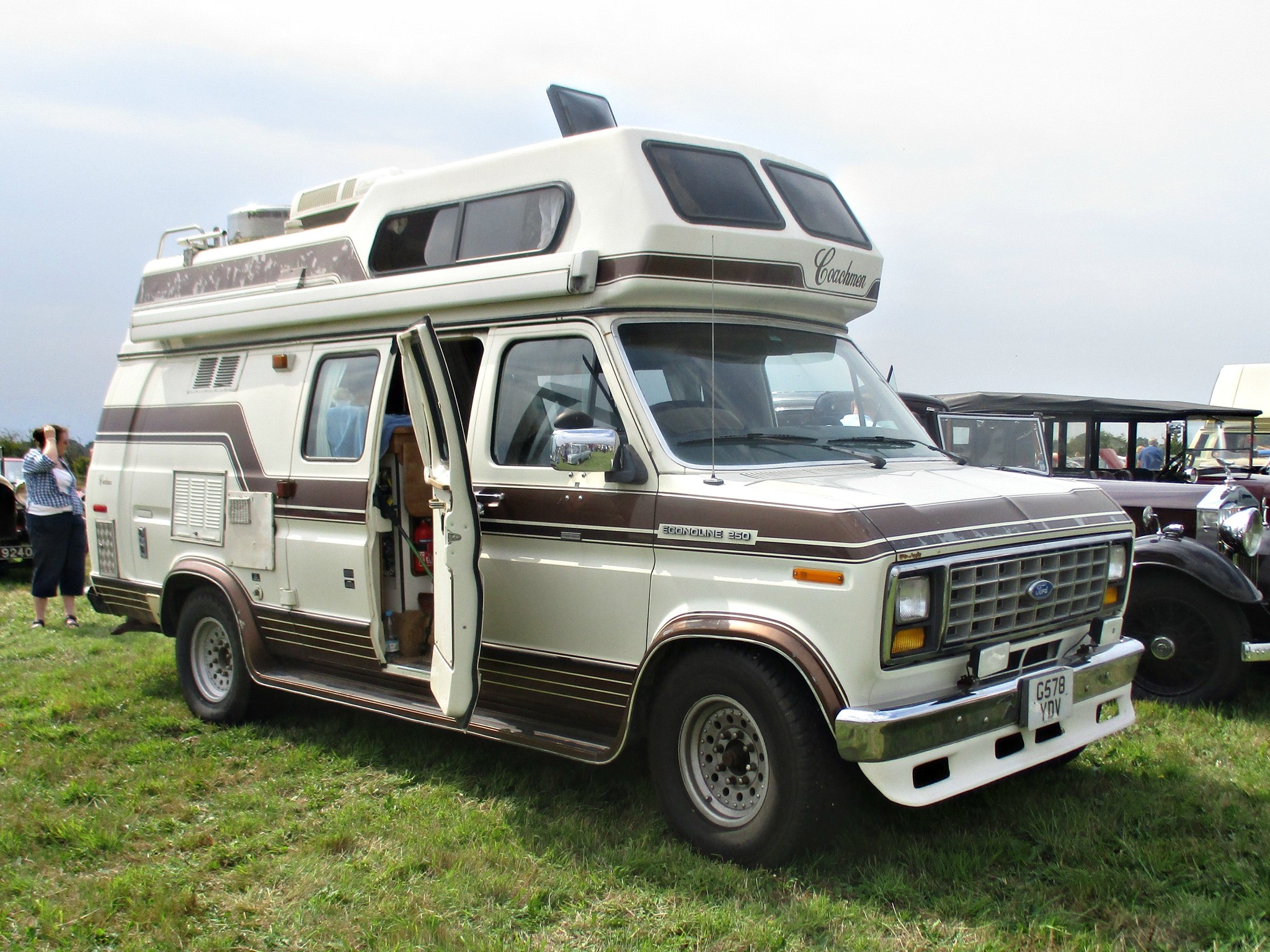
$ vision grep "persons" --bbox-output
[1136,437,1164,471]
[22,424,86,631]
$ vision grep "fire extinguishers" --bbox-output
[413,518,435,572]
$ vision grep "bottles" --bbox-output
[383,611,399,660]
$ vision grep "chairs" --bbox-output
[647,400,745,434]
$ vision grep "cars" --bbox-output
[757,387,1270,695]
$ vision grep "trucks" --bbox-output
[69,87,1146,859]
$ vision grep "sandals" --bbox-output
[30,619,45,628]
[63,615,79,630]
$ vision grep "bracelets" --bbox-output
[45,438,57,442]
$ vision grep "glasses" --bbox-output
[59,439,68,445]
